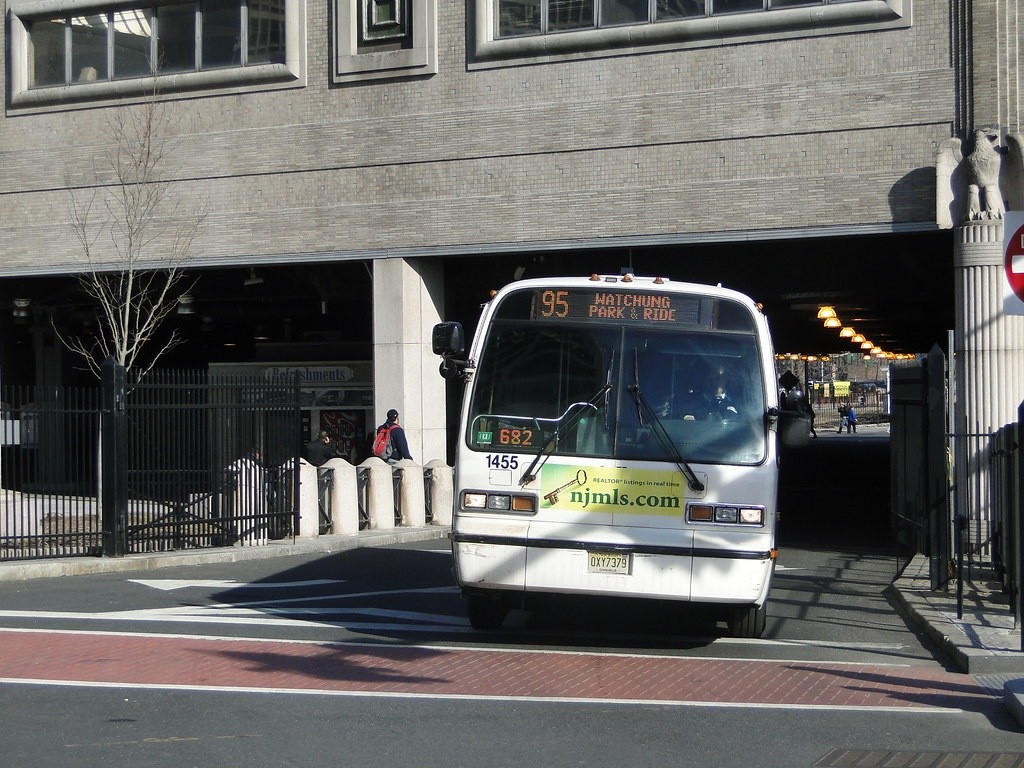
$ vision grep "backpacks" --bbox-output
[372,424,400,459]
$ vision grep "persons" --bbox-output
[836,402,858,434]
[680,367,748,421]
[377,409,413,462]
[305,431,334,467]
[808,404,817,439]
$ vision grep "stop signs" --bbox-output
[1002,211,1024,317]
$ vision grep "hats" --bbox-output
[387,409,398,421]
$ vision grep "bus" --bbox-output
[431,273,810,639]
